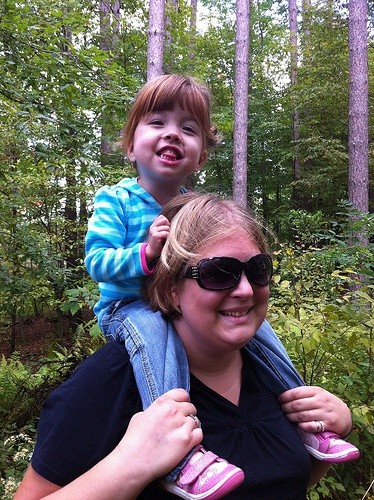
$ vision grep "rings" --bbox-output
[189,414,198,427]
[316,420,324,432]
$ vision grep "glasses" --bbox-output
[182,254,273,291]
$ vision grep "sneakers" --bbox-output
[163,443,244,500]
[296,424,360,463]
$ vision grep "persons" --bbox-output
[82,73,361,500]
[13,193,352,500]
